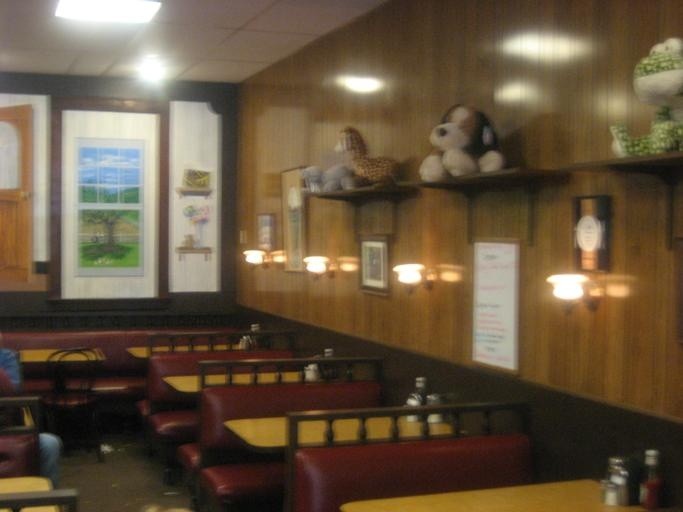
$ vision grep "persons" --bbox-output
[0,347,61,488]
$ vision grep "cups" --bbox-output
[404,393,422,422]
[427,393,440,424]
[303,361,319,381]
[596,459,629,509]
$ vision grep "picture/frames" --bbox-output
[255,213,275,251]
[467,234,521,375]
[279,165,308,273]
[358,233,392,296]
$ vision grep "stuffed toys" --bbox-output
[418,104,507,183]
[611,37,683,157]
[302,125,402,192]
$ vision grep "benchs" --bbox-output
[176,355,382,512]
[0,329,245,396]
[279,400,544,512]
[136,328,321,482]
[0,396,77,511]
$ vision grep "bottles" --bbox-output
[637,448,664,511]
[411,375,427,422]
[322,349,334,378]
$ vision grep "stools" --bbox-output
[39,390,104,464]
